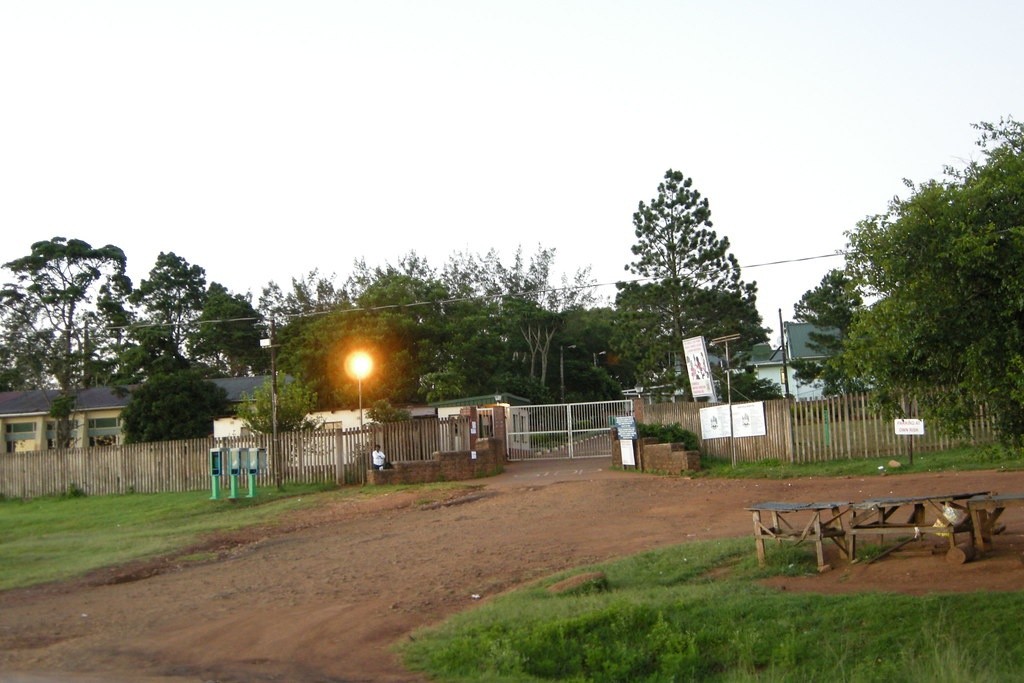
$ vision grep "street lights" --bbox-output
[559,345,576,404]
[711,332,742,469]
[344,351,375,488]
[593,351,607,367]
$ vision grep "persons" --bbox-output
[687,352,709,379]
[372,445,385,470]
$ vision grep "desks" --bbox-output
[744,488,1024,575]
